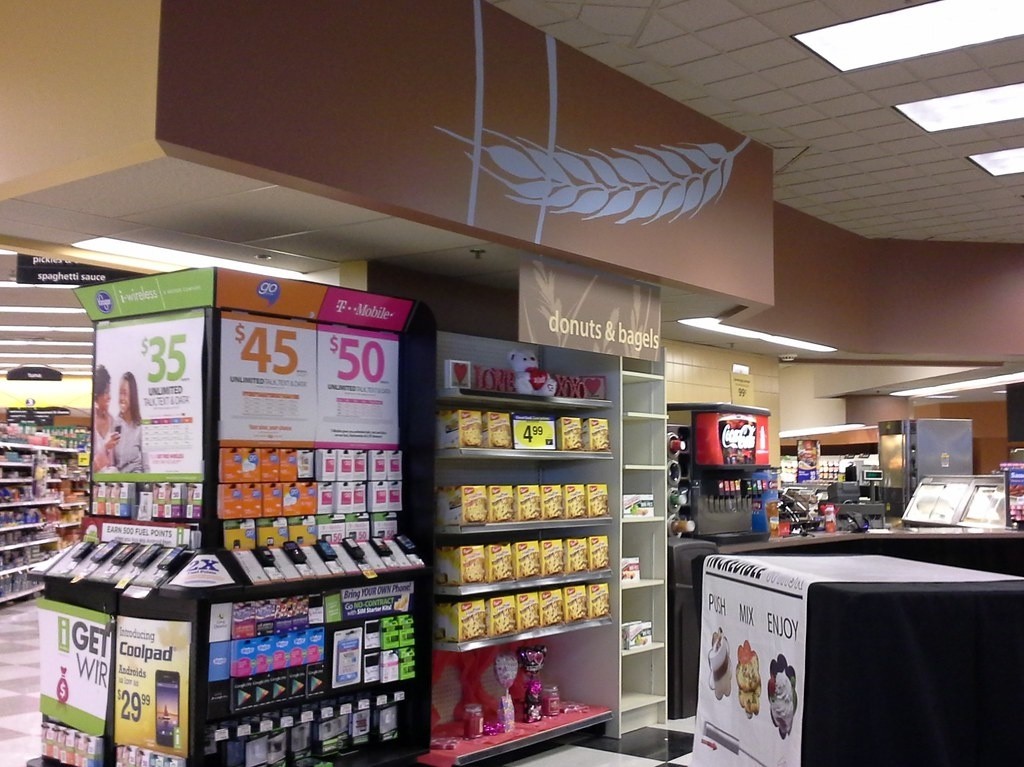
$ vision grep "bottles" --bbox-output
[0,420,91,449]
[825,504,836,534]
[542,685,560,716]
[464,704,483,737]
[670,438,695,533]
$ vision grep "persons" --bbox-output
[93,365,143,473]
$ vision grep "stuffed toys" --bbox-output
[509,351,557,396]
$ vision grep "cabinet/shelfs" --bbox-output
[544,346,670,738]
[57,474,88,553]
[418,333,614,766]
[0,443,78,605]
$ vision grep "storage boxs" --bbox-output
[436,406,611,453]
[621,558,641,582]
[623,493,656,518]
[93,447,406,555]
[620,619,652,649]
[437,485,612,526]
[434,536,610,583]
[436,585,609,644]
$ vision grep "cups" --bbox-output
[720,419,756,466]
[1008,494,1024,523]
[765,500,780,540]
